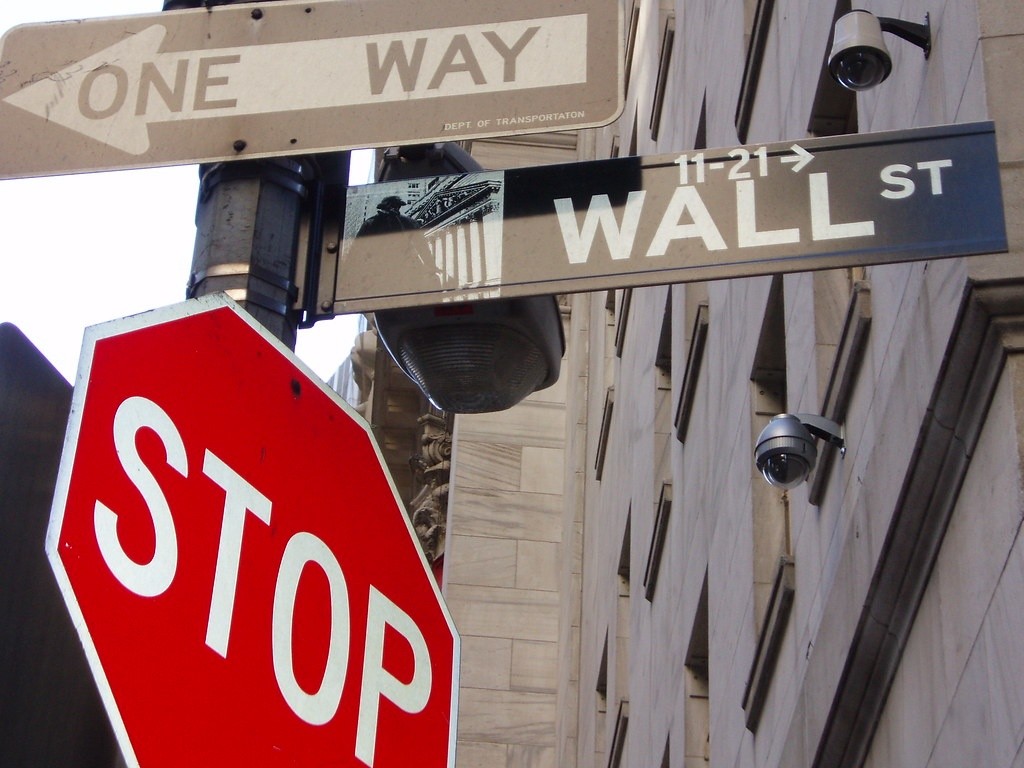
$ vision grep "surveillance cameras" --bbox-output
[752,414,818,489]
[827,9,893,91]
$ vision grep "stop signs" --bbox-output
[44,289,459,768]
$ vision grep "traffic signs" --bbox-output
[1,1,624,180]
[321,121,1013,316]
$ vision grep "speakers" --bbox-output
[371,294,566,415]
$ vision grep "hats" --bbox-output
[377,196,406,209]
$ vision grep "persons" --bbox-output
[340,197,440,310]
